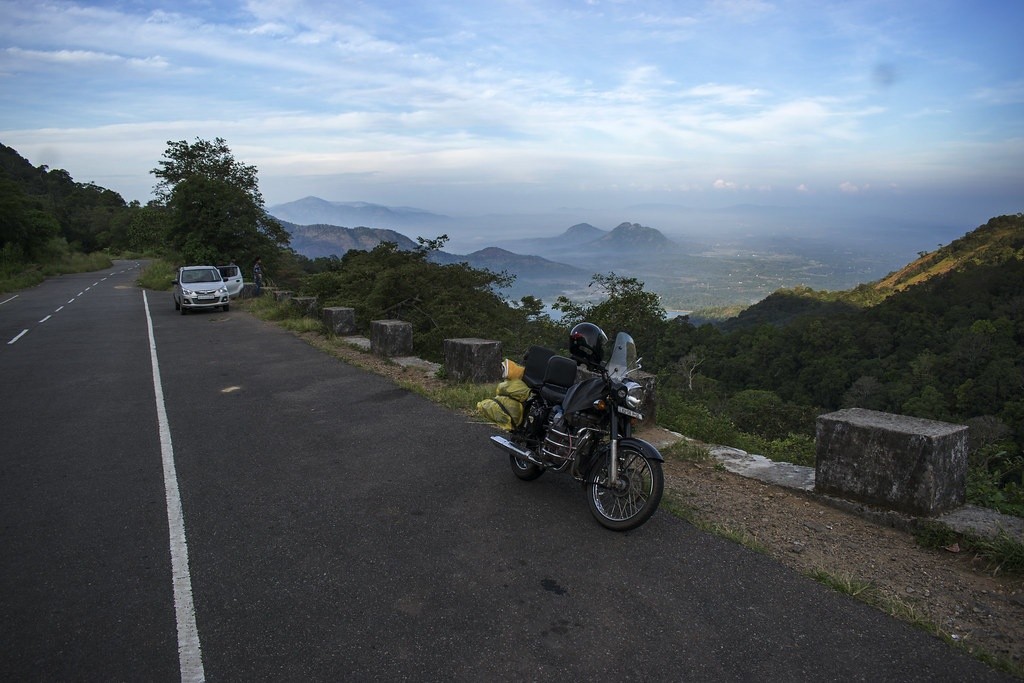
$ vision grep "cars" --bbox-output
[171,266,244,315]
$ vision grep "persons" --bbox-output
[254,255,263,297]
[227,259,237,277]
[218,259,227,277]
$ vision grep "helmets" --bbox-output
[569,322,606,364]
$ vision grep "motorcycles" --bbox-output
[490,332,665,533]
